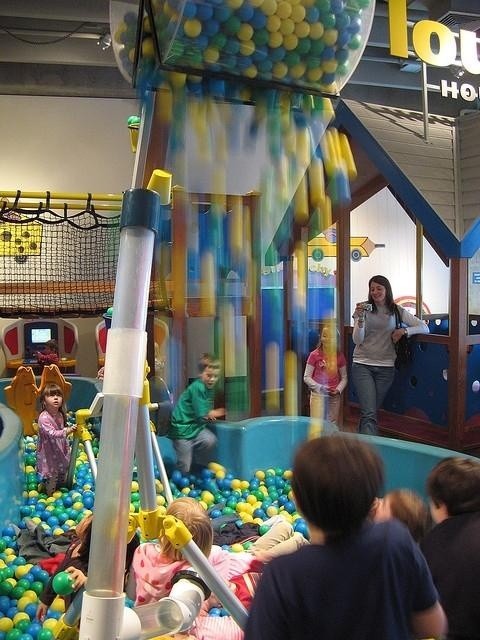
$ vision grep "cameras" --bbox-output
[359,303,373,312]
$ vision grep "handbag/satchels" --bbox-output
[395,335,411,369]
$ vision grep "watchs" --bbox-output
[404,328,408,336]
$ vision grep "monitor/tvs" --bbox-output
[31,328,51,344]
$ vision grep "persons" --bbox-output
[350,275,430,435]
[244,434,451,640]
[35,384,77,497]
[34,511,139,639]
[123,495,308,639]
[418,457,480,639]
[35,340,60,378]
[367,490,434,547]
[303,325,348,431]
[167,356,228,475]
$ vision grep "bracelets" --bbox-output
[358,320,363,323]
